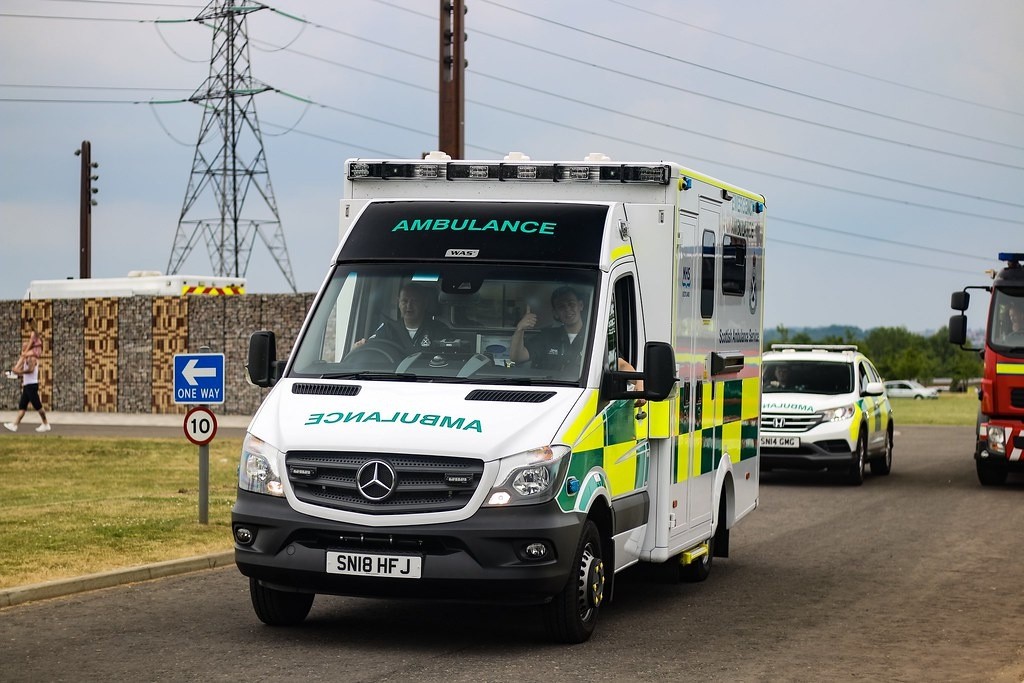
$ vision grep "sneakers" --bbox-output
[4,423,18,432]
[35,423,51,432]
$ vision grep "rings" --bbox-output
[528,318,530,322]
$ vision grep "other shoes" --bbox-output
[7,373,18,379]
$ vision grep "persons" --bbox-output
[7,318,42,379]
[351,285,458,354]
[4,342,51,432]
[768,365,789,388]
[509,285,647,407]
[1002,307,1024,340]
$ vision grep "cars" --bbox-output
[880,379,940,400]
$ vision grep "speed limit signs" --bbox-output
[183,408,218,445]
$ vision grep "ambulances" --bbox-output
[948,251,1024,484]
[232,150,766,644]
[762,342,893,484]
